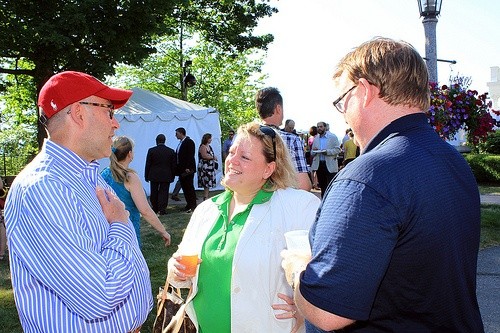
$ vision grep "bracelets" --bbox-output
[291,267,298,292]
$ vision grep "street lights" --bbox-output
[417,0,443,88]
[181,60,197,102]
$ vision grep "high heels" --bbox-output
[312,184,320,190]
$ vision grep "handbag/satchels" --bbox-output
[152,276,197,333]
[215,159,218,170]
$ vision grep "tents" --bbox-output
[112,87,226,197]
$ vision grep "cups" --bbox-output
[178,243,198,277]
[284,230,311,256]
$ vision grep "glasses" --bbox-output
[260,124,277,162]
[67,102,115,120]
[332,82,378,114]
[229,134,233,136]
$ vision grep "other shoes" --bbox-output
[182,207,196,213]
[203,197,207,201]
[159,211,168,215]
[170,195,182,202]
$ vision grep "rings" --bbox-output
[291,310,297,317]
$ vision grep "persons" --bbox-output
[101,136,171,262]
[197,134,217,200]
[168,125,322,333]
[2,70,154,333]
[171,176,199,201]
[281,37,485,333]
[223,130,234,161]
[256,86,314,192]
[144,134,177,215]
[174,127,196,213]
[280,120,358,190]
[310,122,342,200]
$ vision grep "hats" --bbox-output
[38,71,133,125]
